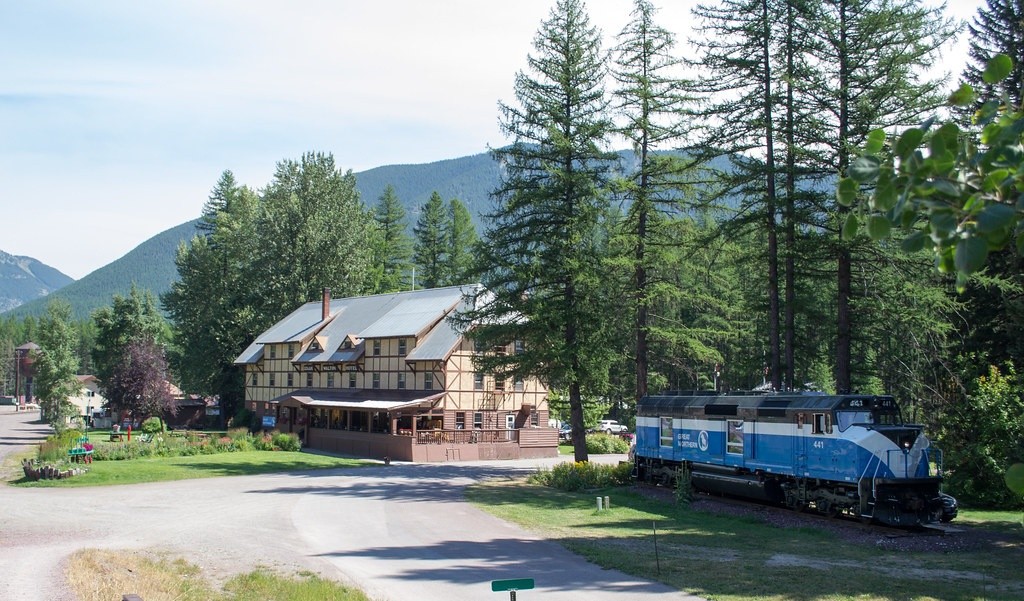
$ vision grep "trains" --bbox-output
[630,391,959,522]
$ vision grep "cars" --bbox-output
[558,424,592,440]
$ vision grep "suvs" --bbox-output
[596,420,628,434]
